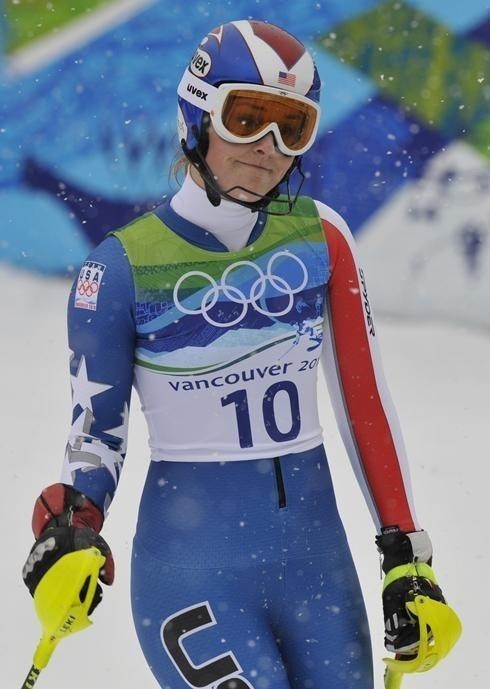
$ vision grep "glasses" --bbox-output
[208,83,321,157]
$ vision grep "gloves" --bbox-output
[375,523,445,657]
[21,484,115,614]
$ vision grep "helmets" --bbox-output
[177,20,321,151]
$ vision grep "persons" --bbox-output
[22,21,462,689]
